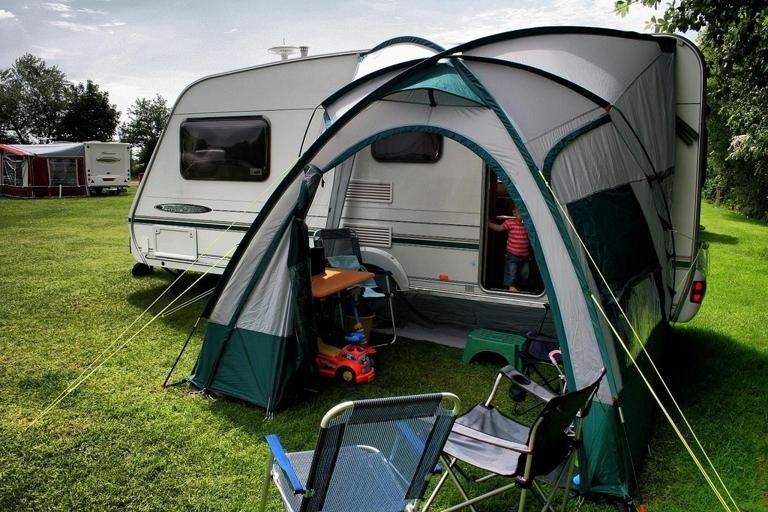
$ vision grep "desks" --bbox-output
[309,267,374,298]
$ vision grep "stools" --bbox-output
[463,328,527,373]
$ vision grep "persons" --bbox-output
[488,208,535,293]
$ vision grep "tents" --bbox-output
[0,141,88,199]
[188,24,672,500]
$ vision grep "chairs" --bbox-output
[313,228,396,350]
[416,365,606,512]
[260,391,460,512]
